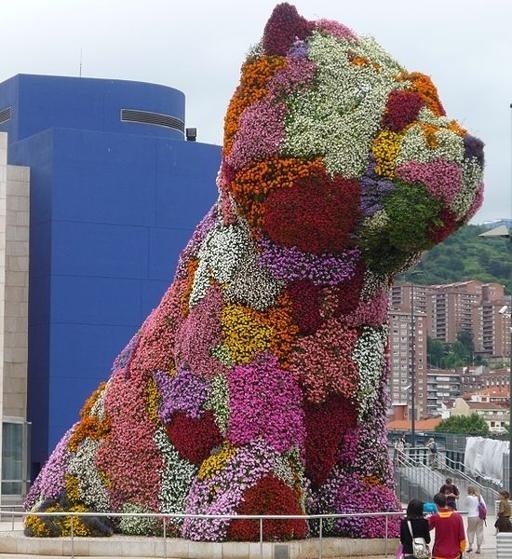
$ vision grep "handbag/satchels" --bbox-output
[478,495,486,520]
[412,537,430,559]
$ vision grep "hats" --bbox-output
[500,490,509,498]
[448,501,457,510]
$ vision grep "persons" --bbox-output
[462,485,486,554]
[494,489,512,532]
[438,478,459,511]
[395,433,407,465]
[428,493,467,559]
[399,498,431,559]
[426,437,437,467]
